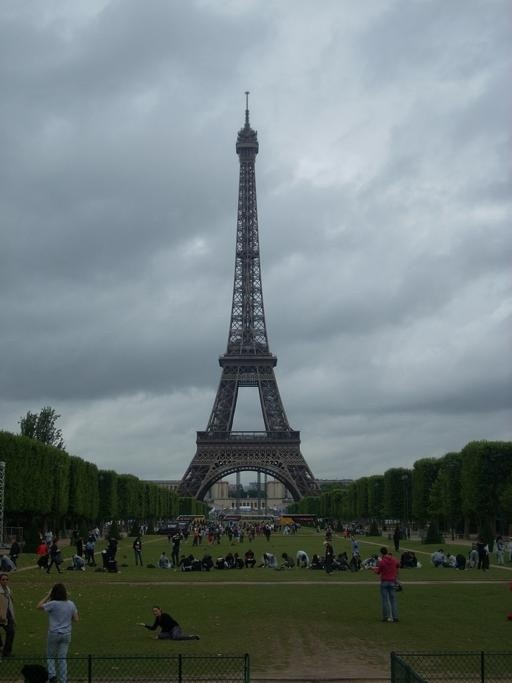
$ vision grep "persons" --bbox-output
[0,573,18,657]
[1,522,145,575]
[155,518,276,571]
[391,522,507,571]
[369,546,402,624]
[135,605,201,641]
[37,582,81,682]
[278,519,383,574]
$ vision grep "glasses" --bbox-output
[1,578,9,581]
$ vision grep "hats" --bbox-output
[323,541,328,545]
[21,664,48,682]
[395,581,402,592]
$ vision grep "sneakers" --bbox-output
[385,618,392,622]
[393,617,401,621]
[190,634,200,640]
[2,652,14,656]
[50,675,57,683]
